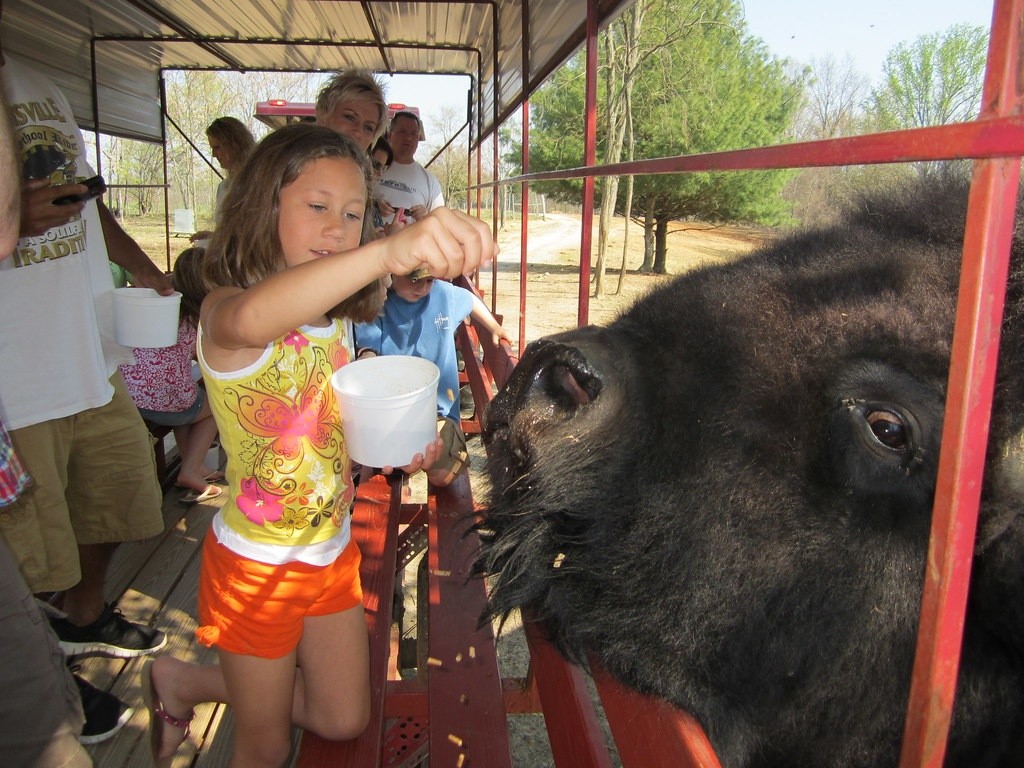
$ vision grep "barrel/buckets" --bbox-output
[331,354,440,468]
[113,288,183,348]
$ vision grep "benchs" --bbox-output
[294,275,722,768]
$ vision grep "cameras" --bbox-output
[53,176,107,206]
[392,207,411,216]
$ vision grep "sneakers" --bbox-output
[48,602,167,657]
[71,674,133,743]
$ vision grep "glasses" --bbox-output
[373,160,388,172]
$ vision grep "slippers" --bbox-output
[140,659,195,768]
[179,484,222,502]
[175,470,222,486]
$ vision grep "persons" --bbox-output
[117,246,226,499]
[1,110,101,768]
[187,116,260,243]
[150,122,502,768]
[0,41,176,745]
[310,68,514,515]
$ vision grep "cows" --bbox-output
[448,169,1024,768]
